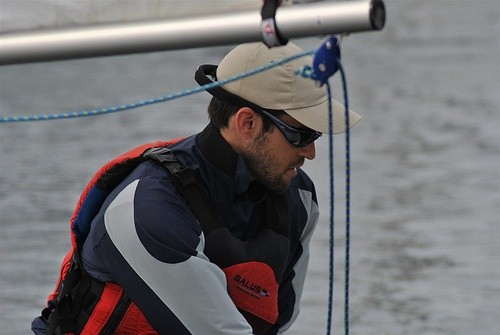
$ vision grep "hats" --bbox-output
[215,38,361,134]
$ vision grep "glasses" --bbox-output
[262,110,322,150]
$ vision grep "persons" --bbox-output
[30,36,360,335]
